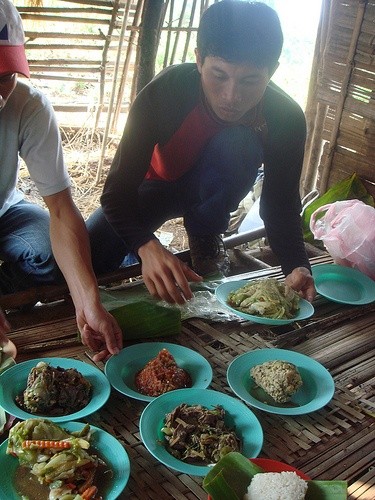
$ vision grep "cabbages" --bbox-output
[6,419,113,500]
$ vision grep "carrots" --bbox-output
[81,486,98,500]
[21,440,71,450]
[77,480,91,493]
[64,482,76,489]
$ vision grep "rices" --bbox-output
[244,471,308,499]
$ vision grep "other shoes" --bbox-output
[186,228,230,279]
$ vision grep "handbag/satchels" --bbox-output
[238,173,375,280]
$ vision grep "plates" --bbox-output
[207,458,312,500]
[311,263,375,305]
[214,280,314,326]
[0,336,16,371]
[226,348,335,416]
[0,421,130,500]
[104,342,213,403]
[139,388,266,475]
[0,357,111,422]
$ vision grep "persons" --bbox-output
[0,0,124,363]
[83,0,316,308]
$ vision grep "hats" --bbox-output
[0,0,32,79]
